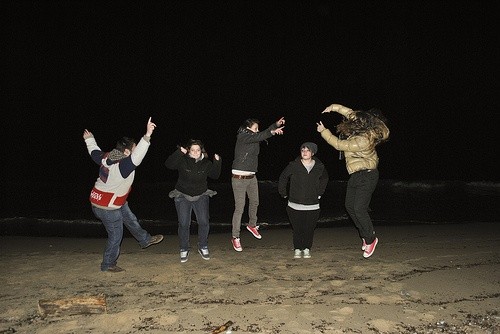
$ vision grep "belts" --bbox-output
[351,169,372,174]
[232,174,255,179]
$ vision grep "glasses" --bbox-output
[301,149,310,152]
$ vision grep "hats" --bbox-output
[300,143,318,154]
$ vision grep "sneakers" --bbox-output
[107,265,125,273]
[303,248,311,258]
[143,234,163,249]
[363,237,378,258]
[180,251,190,263]
[362,231,375,251]
[198,246,210,260]
[232,237,242,252]
[294,249,302,259]
[246,225,262,239]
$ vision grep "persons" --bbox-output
[316,104,390,259]
[278,142,328,258]
[164,140,223,262]
[232,116,285,251]
[83,116,165,273]
